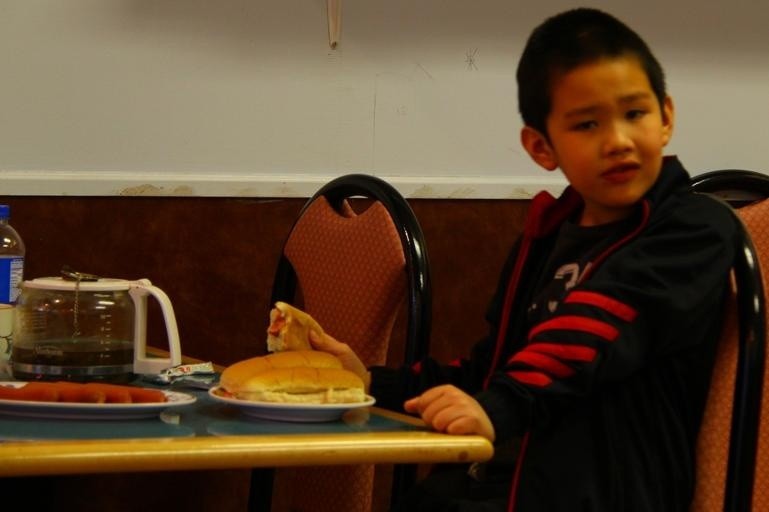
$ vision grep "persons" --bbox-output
[309,8,737,512]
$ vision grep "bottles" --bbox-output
[0,204,26,382]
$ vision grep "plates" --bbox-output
[0,377,198,423]
[206,382,376,423]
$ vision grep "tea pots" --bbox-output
[4,270,182,387]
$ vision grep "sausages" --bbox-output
[0,381,168,403]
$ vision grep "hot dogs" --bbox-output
[216,301,365,404]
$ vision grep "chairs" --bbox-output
[242,172,442,511]
[671,165,768,510]
